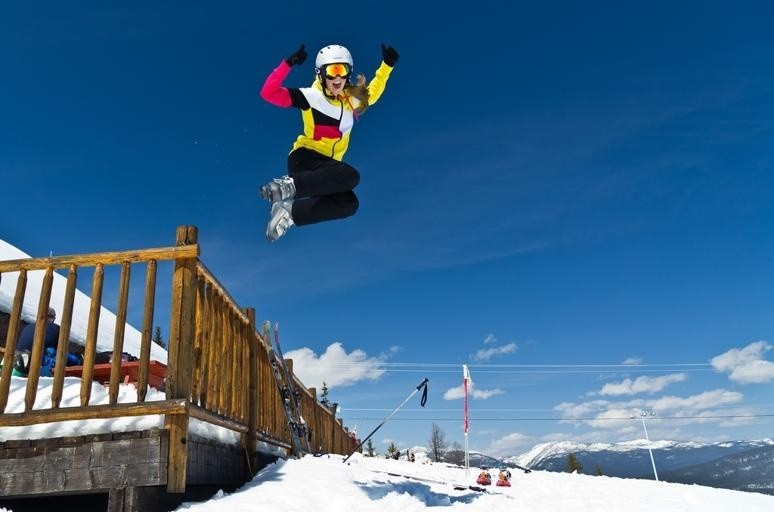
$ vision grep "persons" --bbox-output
[16,306,61,377]
[395,450,400,459]
[388,452,395,459]
[258,43,399,243]
[410,454,415,462]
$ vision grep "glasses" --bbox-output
[316,63,352,80]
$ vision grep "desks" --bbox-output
[52,360,167,388]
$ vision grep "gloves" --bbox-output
[382,44,399,67]
[286,44,307,66]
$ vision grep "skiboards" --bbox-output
[263,318,313,458]
[477,465,510,487]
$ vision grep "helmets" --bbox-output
[316,45,353,73]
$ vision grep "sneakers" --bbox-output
[266,200,295,240]
[267,175,296,202]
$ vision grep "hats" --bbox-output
[47,307,55,316]
